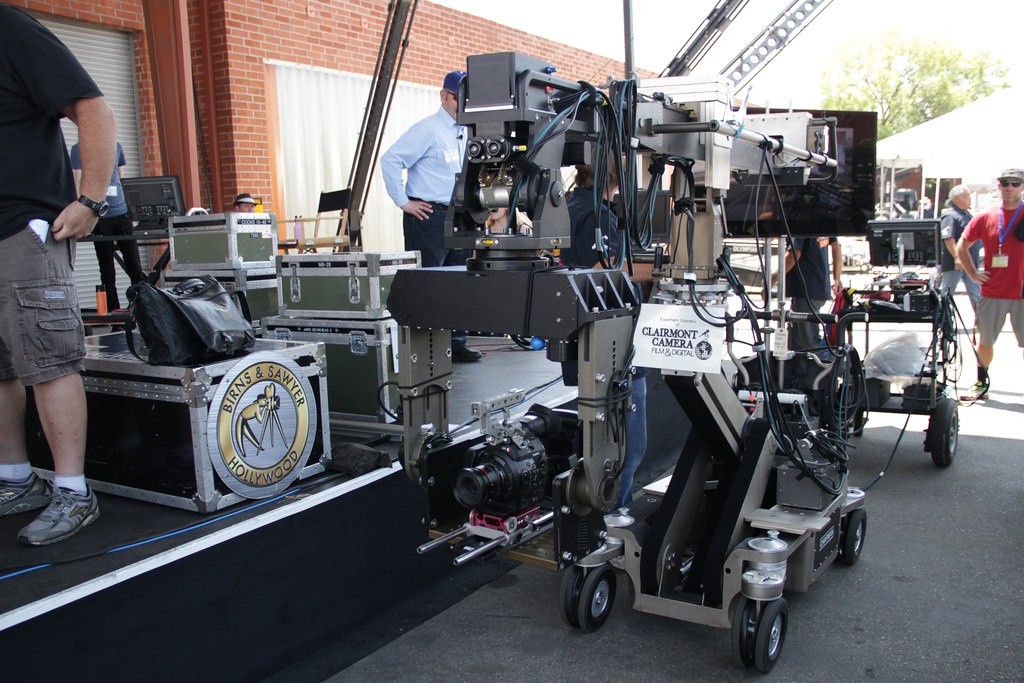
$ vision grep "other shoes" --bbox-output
[451,339,482,362]
[618,509,630,515]
[604,513,635,528]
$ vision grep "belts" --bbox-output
[408,196,449,210]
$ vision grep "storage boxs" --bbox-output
[168,212,279,270]
[165,268,278,328]
[276,250,422,319]
[262,316,399,424]
[31,330,335,514]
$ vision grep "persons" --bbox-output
[760,236,843,417]
[956,167,1024,400]
[380,70,479,363]
[71,140,148,332]
[485,207,533,237]
[560,142,671,527]
[232,193,255,212]
[941,185,980,315]
[0,3,117,547]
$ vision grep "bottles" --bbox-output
[294,215,305,242]
[95,284,107,316]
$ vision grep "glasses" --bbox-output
[999,180,1021,187]
[447,91,458,101]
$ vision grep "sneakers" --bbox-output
[0,471,52,515]
[960,380,989,401]
[17,478,100,545]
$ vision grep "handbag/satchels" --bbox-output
[123,274,256,368]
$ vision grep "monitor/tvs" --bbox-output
[120,176,186,222]
[867,219,942,267]
[693,108,878,236]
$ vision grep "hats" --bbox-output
[997,167,1024,179]
[443,71,467,94]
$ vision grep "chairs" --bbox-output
[278,186,351,254]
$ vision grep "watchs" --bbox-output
[955,258,962,265]
[77,192,110,219]
